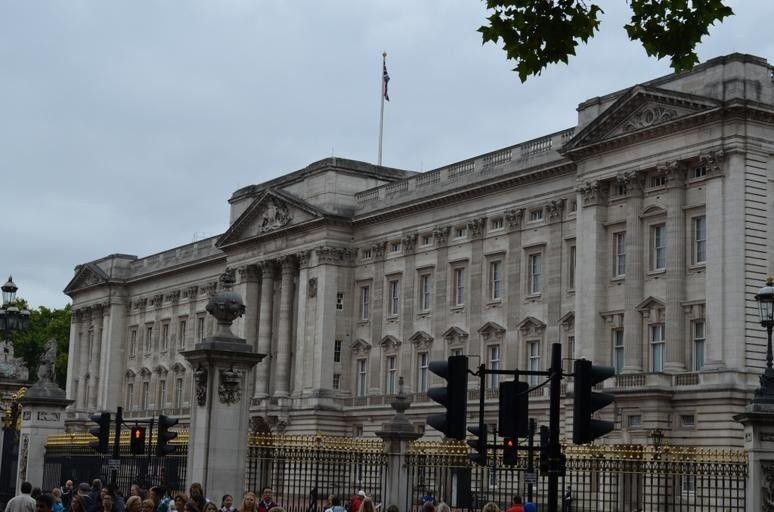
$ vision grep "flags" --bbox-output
[383,63,390,101]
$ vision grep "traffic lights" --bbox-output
[130,426,144,455]
[86,411,110,452]
[427,355,468,439]
[466,423,487,466]
[572,357,614,442]
[154,414,178,457]
[503,438,518,465]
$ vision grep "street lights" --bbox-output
[750,277,773,404]
[0,275,30,361]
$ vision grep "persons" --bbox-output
[323,488,537,512]
[565,486,572,512]
[5,479,288,512]
